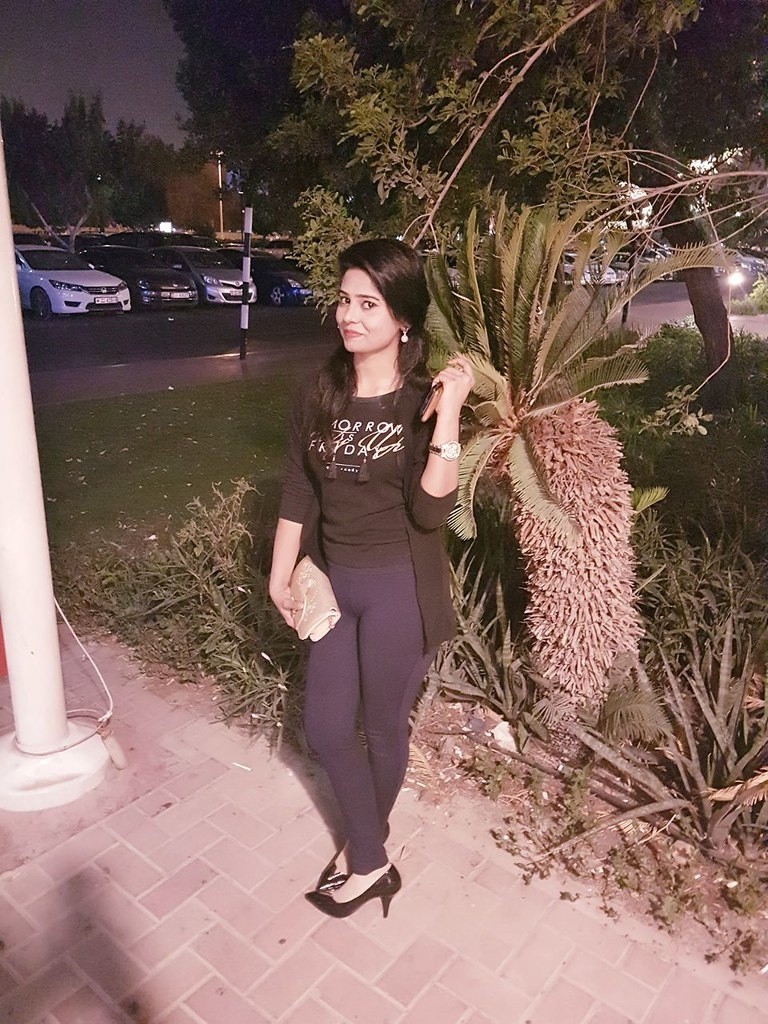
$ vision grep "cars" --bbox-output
[548,244,768,289]
[61,246,200,311]
[145,245,259,305]
[12,225,216,250]
[213,248,318,309]
[13,244,132,318]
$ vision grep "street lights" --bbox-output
[211,149,224,239]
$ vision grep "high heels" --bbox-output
[304,864,402,918]
[316,821,390,892]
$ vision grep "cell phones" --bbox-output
[418,381,442,416]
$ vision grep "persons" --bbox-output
[268,239,476,918]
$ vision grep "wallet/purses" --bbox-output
[290,555,342,642]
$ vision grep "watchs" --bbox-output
[429,439,463,461]
